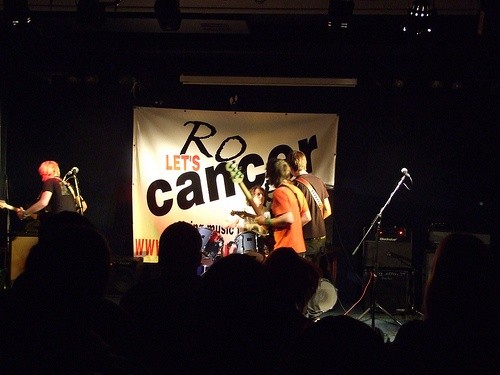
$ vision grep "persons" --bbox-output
[286,150,333,319]
[0,210,500,375]
[17,160,87,223]
[254,158,312,256]
[226,185,271,228]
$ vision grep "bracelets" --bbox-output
[265,218,272,224]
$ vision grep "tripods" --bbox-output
[351,175,408,334]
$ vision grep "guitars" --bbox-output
[224,162,275,236]
[0,199,40,221]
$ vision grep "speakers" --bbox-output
[359,237,412,315]
[8,232,39,280]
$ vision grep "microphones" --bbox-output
[401,167,412,180]
[68,166,79,175]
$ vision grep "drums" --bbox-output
[234,232,266,264]
[307,278,338,313]
[197,228,225,257]
